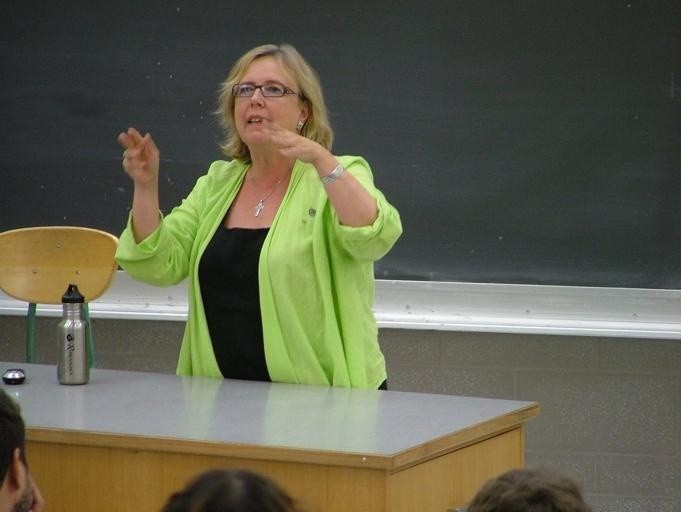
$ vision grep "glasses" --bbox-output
[231,82,300,97]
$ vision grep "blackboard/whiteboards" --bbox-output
[0,0,681,341]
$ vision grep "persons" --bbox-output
[113,42,404,391]
[462,465,593,511]
[160,467,296,512]
[0,387,45,512]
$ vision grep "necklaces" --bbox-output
[247,166,290,217]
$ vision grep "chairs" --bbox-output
[0,226,120,368]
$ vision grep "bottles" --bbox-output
[56,284,90,385]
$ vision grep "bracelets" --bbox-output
[321,162,344,185]
